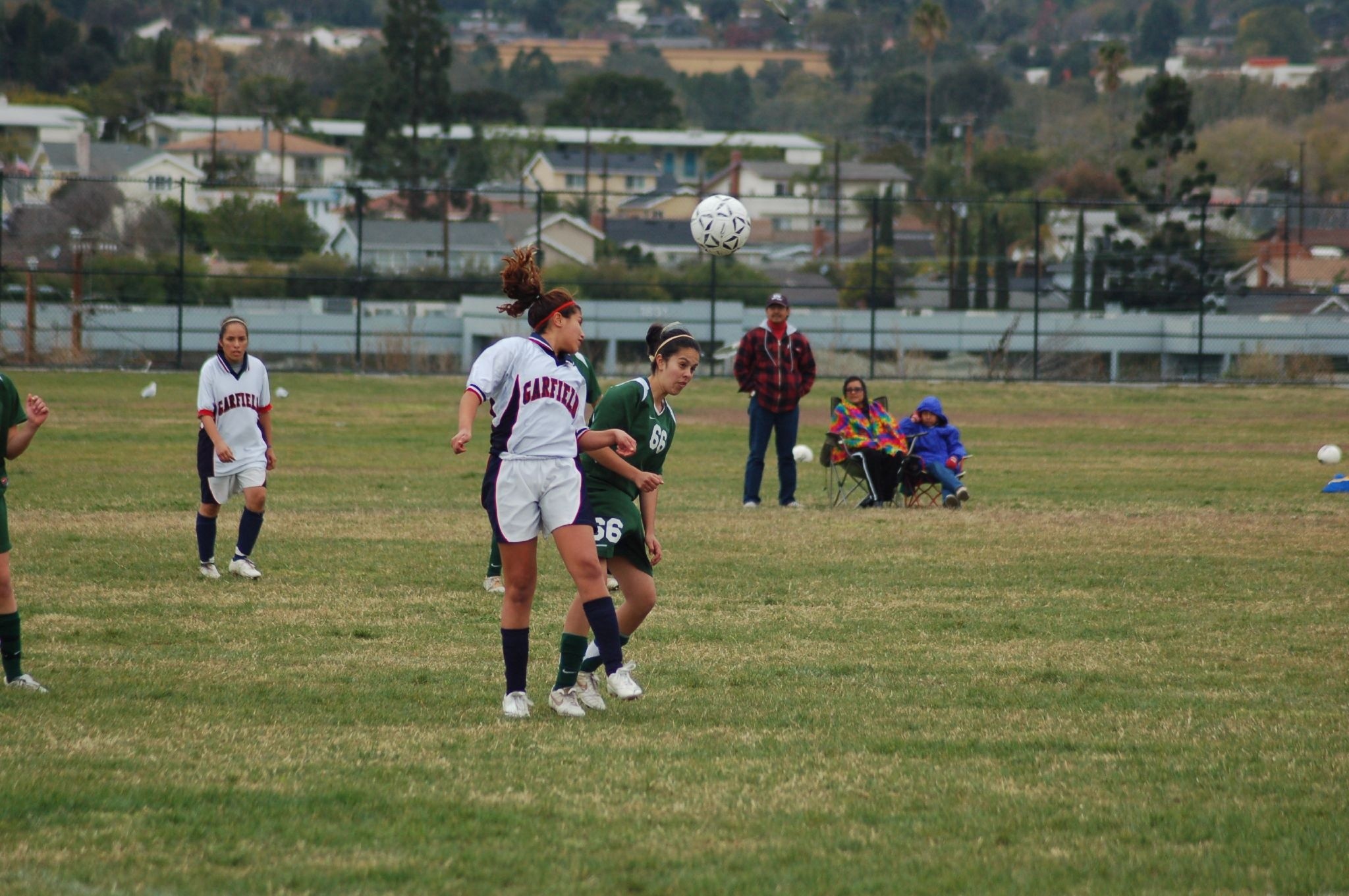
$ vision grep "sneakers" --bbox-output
[199,560,220,581]
[228,557,261,579]
[547,684,585,717]
[604,660,642,700]
[574,670,606,711]
[607,579,620,592]
[483,574,506,594]
[502,688,535,719]
[3,674,48,695]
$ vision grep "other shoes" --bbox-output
[786,501,801,508]
[957,488,969,502]
[743,500,756,508]
[946,494,962,510]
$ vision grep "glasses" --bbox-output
[846,387,864,393]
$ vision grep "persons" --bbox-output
[449,241,639,719]
[481,347,604,596]
[196,316,279,581]
[895,394,971,510]
[828,376,922,513]
[1,367,54,693]
[574,318,704,712]
[728,293,819,510]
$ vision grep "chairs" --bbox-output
[819,395,974,510]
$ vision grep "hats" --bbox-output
[767,293,788,307]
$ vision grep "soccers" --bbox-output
[1316,443,1343,464]
[689,193,752,257]
[792,444,813,463]
